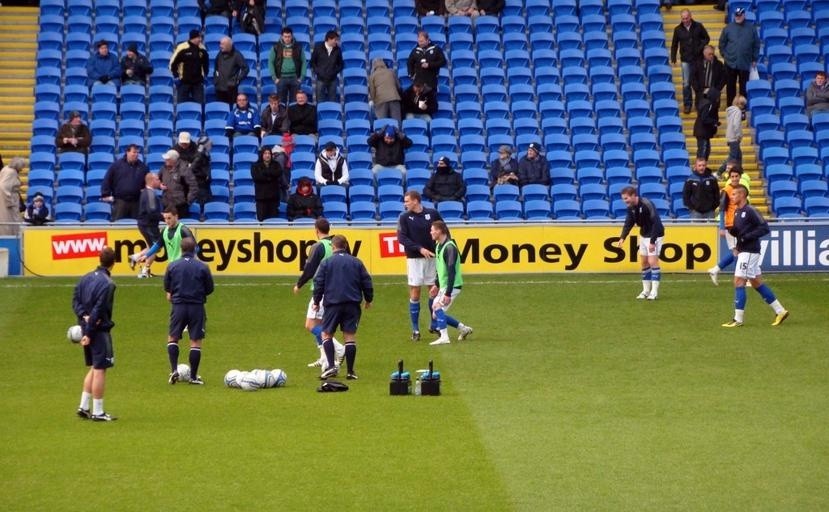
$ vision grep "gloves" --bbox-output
[376,124,388,137]
[394,127,404,139]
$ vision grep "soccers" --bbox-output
[67,325,84,343]
[176,365,191,382]
[224,369,287,391]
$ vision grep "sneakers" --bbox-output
[137,270,153,279]
[648,291,658,299]
[637,291,648,300]
[129,254,137,272]
[412,330,420,341]
[428,337,449,346]
[428,327,439,333]
[708,268,719,286]
[772,310,789,326]
[458,328,472,341]
[76,408,91,417]
[307,347,358,379]
[92,411,117,421]
[712,171,725,181]
[168,371,179,385]
[189,378,203,384]
[722,318,744,327]
[684,107,690,114]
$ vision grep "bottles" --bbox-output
[389,371,413,396]
[416,378,421,396]
[421,372,440,395]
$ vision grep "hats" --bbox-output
[529,143,541,154]
[735,7,745,17]
[162,150,178,161]
[497,145,511,153]
[281,132,295,169]
[384,127,395,137]
[439,157,449,165]
[179,131,190,143]
[190,30,198,39]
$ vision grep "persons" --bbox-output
[429,220,472,345]
[721,185,790,328]
[617,186,664,300]
[71,247,117,421]
[293,218,345,363]
[805,72,829,115]
[709,167,752,286]
[137,207,207,338]
[164,237,214,386]
[312,235,373,381]
[1,1,553,279]
[398,191,448,342]
[661,2,762,221]
